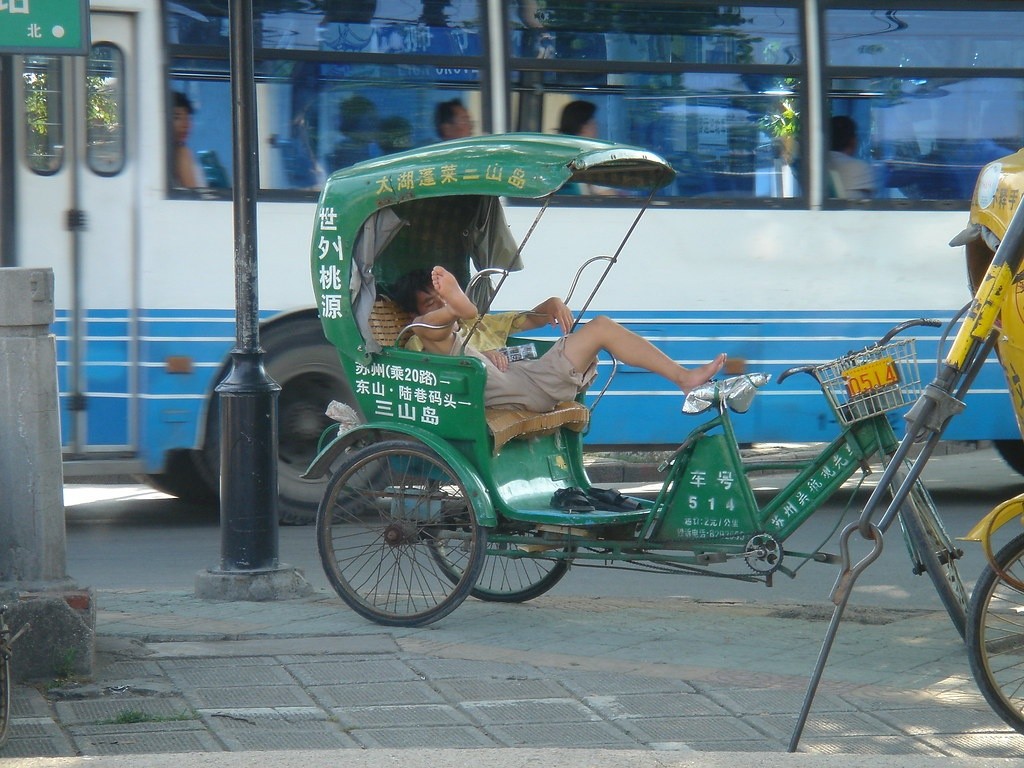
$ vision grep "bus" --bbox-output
[2,0,1024,523]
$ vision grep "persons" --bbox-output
[933,119,1017,200]
[167,93,208,187]
[397,266,726,418]
[827,119,891,200]
[557,99,619,196]
[436,99,475,141]
[377,117,413,154]
[325,97,378,176]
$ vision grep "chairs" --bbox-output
[918,150,971,200]
[788,161,845,200]
[370,300,592,453]
[196,150,230,188]
[270,136,325,192]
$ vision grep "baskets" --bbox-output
[813,338,922,426]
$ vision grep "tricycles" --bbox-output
[945,148,1023,736]
[299,132,972,646]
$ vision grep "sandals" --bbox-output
[550,487,595,512]
[586,487,643,512]
